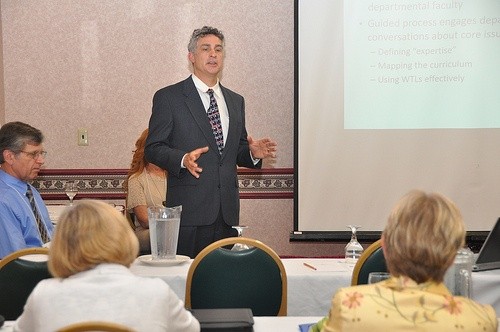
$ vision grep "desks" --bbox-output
[125,255,500,332]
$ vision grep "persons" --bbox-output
[145,26,276,258]
[307,189,500,332]
[0,120,55,263]
[0,199,201,332]
[128,127,168,256]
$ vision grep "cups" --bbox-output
[368,272,390,284]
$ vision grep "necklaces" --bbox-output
[147,167,166,206]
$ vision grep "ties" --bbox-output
[206,89,225,155]
[26,184,51,243]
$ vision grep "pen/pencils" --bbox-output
[304,262,317,271]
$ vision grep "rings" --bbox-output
[267,148,270,152]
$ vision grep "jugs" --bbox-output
[147,205,183,260]
[445,248,474,299]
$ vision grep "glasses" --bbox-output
[4,145,47,159]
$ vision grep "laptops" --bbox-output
[470,217,500,271]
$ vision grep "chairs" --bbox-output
[0,245,54,321]
[184,237,288,317]
[351,237,390,287]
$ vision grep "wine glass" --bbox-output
[65,183,78,207]
[230,226,250,251]
[345,226,364,265]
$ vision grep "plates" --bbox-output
[137,253,191,266]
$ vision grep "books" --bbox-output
[189,307,255,332]
